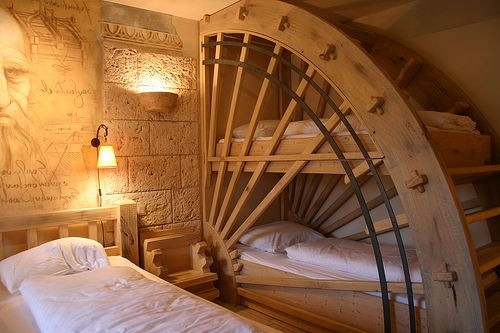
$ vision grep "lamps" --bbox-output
[138,74,179,114]
[90,124,118,208]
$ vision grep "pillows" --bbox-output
[237,220,325,254]
[0,237,109,294]
[233,120,282,138]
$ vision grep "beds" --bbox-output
[0,208,284,333]
[201,112,500,333]
